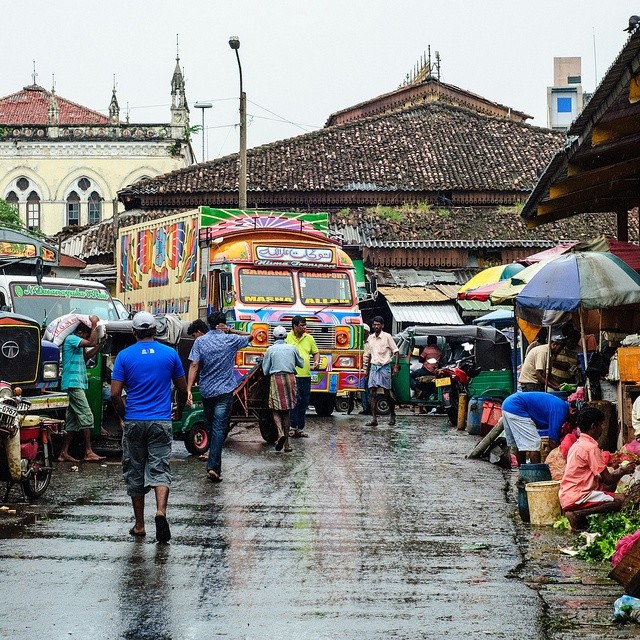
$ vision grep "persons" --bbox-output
[502,391,590,470]
[544,422,610,480]
[111,310,188,543]
[450,343,469,370]
[284,316,320,437]
[359,323,377,415]
[524,327,548,358]
[363,316,399,426]
[558,407,636,534]
[632,396,640,443]
[261,325,304,452]
[186,312,254,482]
[102,356,127,427]
[57,314,108,462]
[410,335,443,399]
[546,330,582,393]
[517,334,566,392]
[187,319,209,340]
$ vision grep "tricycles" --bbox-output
[376,326,514,414]
[84,320,210,455]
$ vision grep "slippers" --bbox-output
[129,525,146,537]
[284,447,292,451]
[155,515,171,541]
[207,475,223,482]
[274,435,286,451]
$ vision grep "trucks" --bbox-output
[1,276,120,328]
[114,205,363,415]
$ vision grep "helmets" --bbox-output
[466,362,481,377]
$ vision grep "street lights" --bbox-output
[228,35,248,207]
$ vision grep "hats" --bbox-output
[131,311,157,330]
[273,325,287,338]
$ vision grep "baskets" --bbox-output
[0,398,31,439]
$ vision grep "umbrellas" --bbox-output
[487,255,566,393]
[519,233,640,271]
[456,261,526,391]
[515,248,640,401]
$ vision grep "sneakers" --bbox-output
[296,431,308,437]
[565,511,580,531]
[388,413,396,425]
[416,389,422,399]
[289,429,295,437]
[366,421,378,426]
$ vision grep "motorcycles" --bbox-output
[0,379,67,499]
